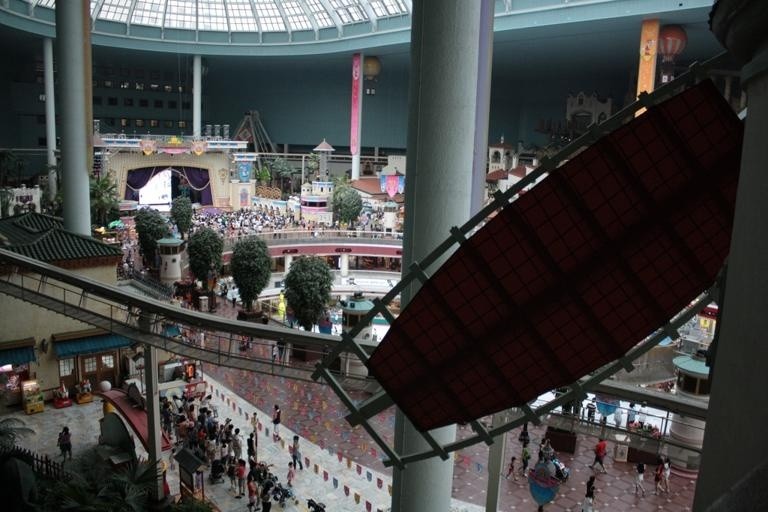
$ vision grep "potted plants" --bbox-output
[333,187,362,236]
[281,255,332,361]
[229,237,270,325]
[169,196,194,243]
[185,227,224,312]
[133,207,165,279]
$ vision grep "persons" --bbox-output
[57,426,73,463]
[239,335,253,355]
[193,202,383,237]
[272,341,293,367]
[585,396,597,423]
[168,281,185,299]
[588,436,608,474]
[582,475,600,512]
[614,400,647,427]
[506,438,545,481]
[220,285,237,308]
[123,249,145,281]
[319,316,332,334]
[633,454,672,496]
[161,393,303,512]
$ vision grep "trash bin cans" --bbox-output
[199,296,209,312]
